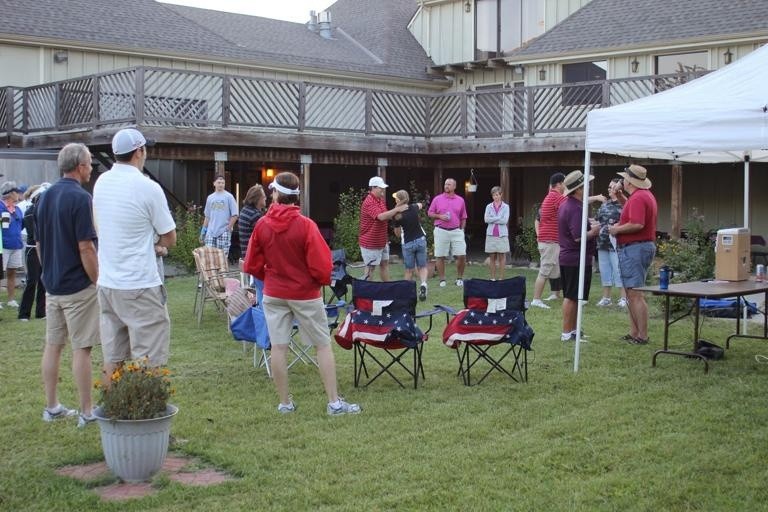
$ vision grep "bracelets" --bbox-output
[200,227,207,240]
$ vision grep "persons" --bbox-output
[392,190,428,301]
[0,182,52,322]
[428,178,467,287]
[238,184,267,261]
[359,176,409,282]
[198,175,238,288]
[484,186,510,281]
[607,164,657,344]
[531,172,630,308]
[557,170,601,343]
[244,172,363,416]
[33,143,100,428]
[93,128,177,398]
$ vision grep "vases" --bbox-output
[91,403,180,485]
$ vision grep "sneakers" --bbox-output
[7,300,19,308]
[440,280,446,287]
[456,278,464,286]
[279,400,296,414]
[419,282,427,301]
[43,404,78,421]
[78,405,98,427]
[328,396,361,415]
[530,293,649,345]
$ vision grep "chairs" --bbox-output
[192,246,243,325]
[321,250,347,301]
[232,276,345,380]
[223,275,258,353]
[443,275,535,386]
[335,277,443,390]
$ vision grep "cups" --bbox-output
[659,265,673,290]
[446,212,450,220]
[2,212,11,228]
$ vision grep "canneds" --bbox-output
[447,211,450,220]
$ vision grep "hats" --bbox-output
[268,174,300,196]
[550,165,653,199]
[113,129,156,155]
[3,182,52,202]
[368,177,389,189]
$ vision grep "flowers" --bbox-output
[94,356,176,418]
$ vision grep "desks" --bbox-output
[627,279,768,374]
